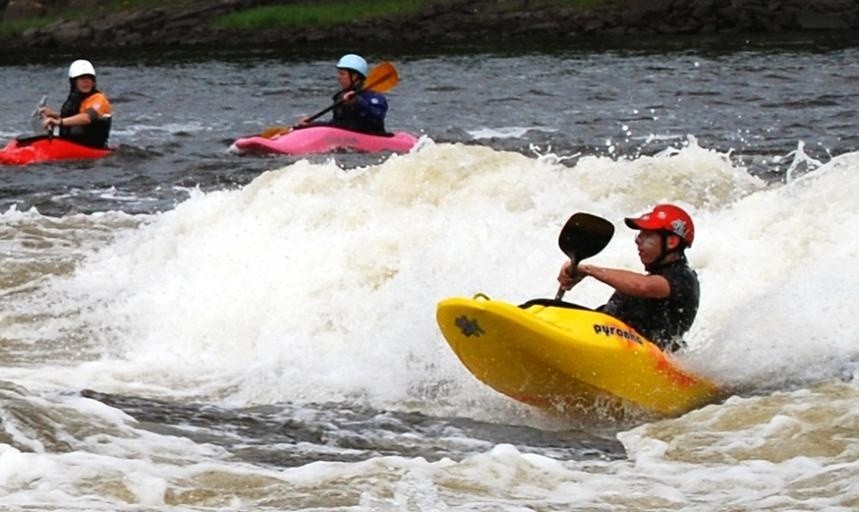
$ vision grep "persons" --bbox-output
[556,204,702,358]
[293,53,389,135]
[37,59,112,148]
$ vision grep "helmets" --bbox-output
[67,59,96,80]
[624,202,695,248]
[335,52,369,78]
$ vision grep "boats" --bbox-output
[234,125,421,157]
[0,134,120,168]
[436,293,739,426]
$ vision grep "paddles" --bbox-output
[556,212,615,301]
[32,95,53,137]
[259,61,400,138]
